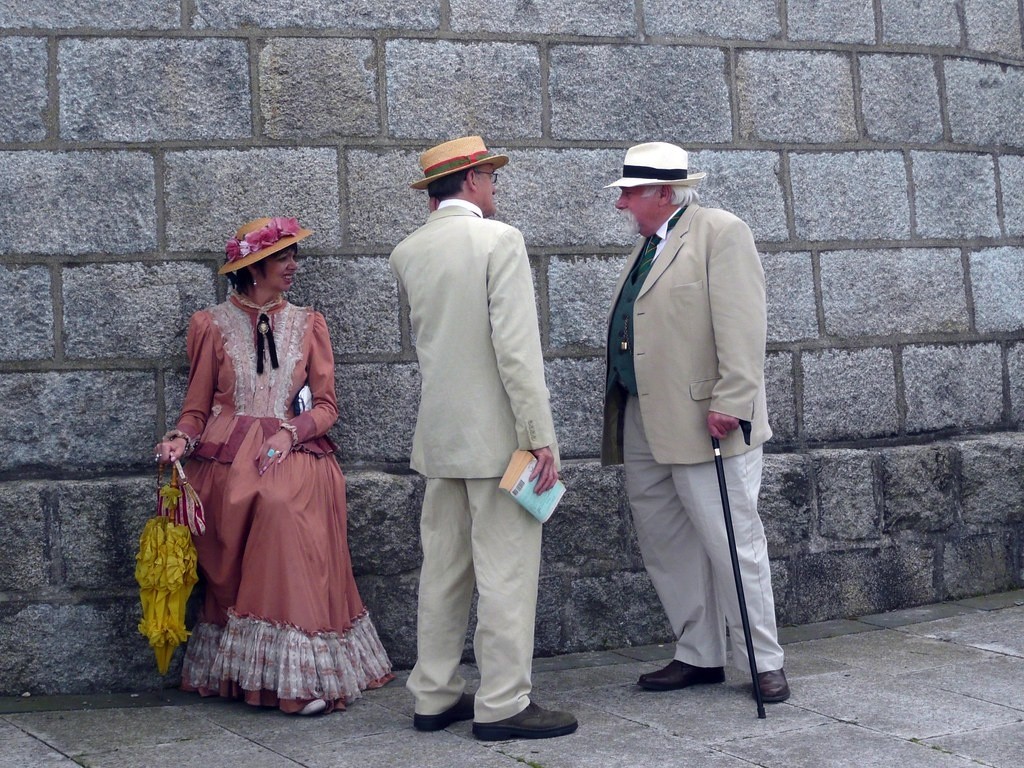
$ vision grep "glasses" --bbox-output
[463,170,498,183]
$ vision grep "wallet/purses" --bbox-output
[293,383,313,417]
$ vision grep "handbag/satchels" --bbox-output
[156,458,207,538]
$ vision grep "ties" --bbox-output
[634,203,688,285]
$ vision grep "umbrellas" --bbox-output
[134,462,200,700]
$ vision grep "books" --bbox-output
[497,448,567,524]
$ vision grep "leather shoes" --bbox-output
[636,658,725,691]
[753,668,790,703]
[471,699,578,741]
[413,692,475,731]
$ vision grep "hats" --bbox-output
[217,216,312,275]
[603,141,706,188]
[409,136,509,190]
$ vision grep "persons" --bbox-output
[599,142,792,703]
[152,217,397,716]
[386,136,578,741]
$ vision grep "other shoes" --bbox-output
[298,698,326,716]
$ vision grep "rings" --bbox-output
[268,449,274,457]
[154,458,158,462]
[155,453,160,457]
[159,453,162,456]
[275,450,280,454]
